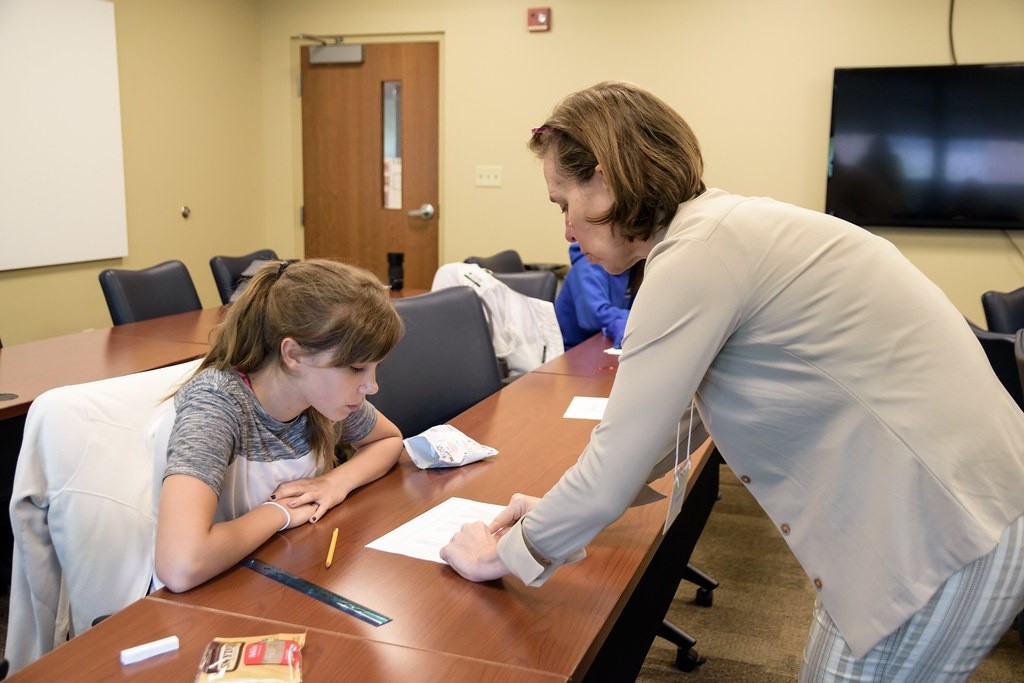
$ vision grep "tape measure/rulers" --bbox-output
[239,555,392,628]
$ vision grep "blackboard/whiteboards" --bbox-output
[0,0,130,272]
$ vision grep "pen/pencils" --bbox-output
[325,526,340,570]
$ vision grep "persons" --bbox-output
[155,259,405,593]
[439,81,1024,683]
[554,242,646,352]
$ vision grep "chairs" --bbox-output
[962,287,1024,411]
[4,357,207,680]
[98,260,203,326]
[367,250,718,670]
[210,249,279,307]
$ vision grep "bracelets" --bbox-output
[261,502,291,531]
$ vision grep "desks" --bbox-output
[0,285,431,421]
[0,331,719,683]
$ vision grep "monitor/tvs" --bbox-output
[823,61,1024,231]
